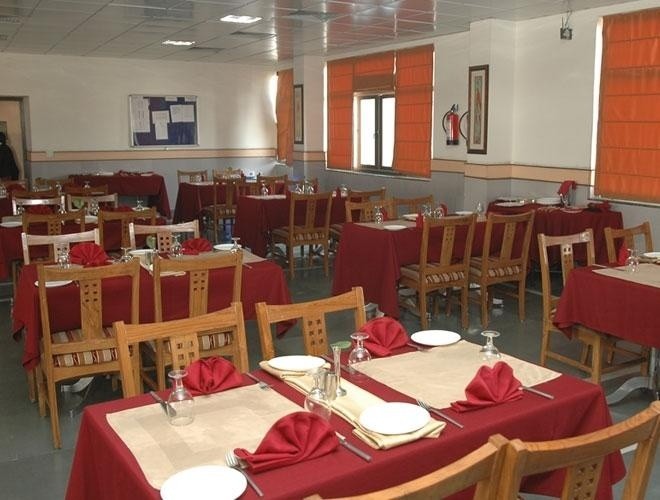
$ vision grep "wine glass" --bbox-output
[304,368,331,420]
[625,249,639,274]
[375,206,383,226]
[260,183,348,197]
[348,332,373,383]
[0,181,145,216]
[479,330,501,369]
[166,369,196,426]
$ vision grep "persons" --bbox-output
[1,131,19,180]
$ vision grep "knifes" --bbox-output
[321,353,356,376]
[592,263,625,272]
[150,390,176,417]
[334,430,371,463]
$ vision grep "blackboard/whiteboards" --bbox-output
[128,94,200,149]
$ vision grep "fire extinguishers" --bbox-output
[442,104,459,145]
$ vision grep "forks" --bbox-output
[416,398,464,429]
[223,447,265,497]
[244,371,275,392]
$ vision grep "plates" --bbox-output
[410,330,462,346]
[267,355,326,372]
[0,207,242,288]
[382,198,562,232]
[643,252,660,258]
[160,466,247,500]
[360,402,430,435]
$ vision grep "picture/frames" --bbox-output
[466,64,489,154]
[293,84,304,144]
